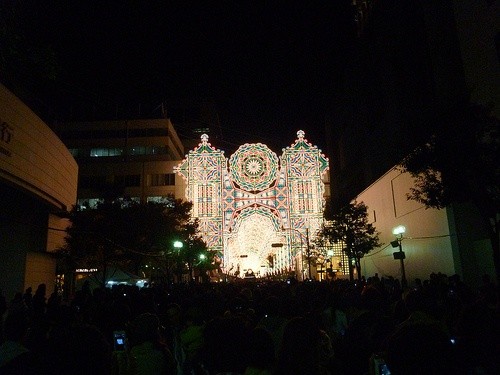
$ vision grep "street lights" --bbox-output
[191,254,205,287]
[171,240,183,283]
[390,225,406,288]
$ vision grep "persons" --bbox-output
[0,270,500,375]
